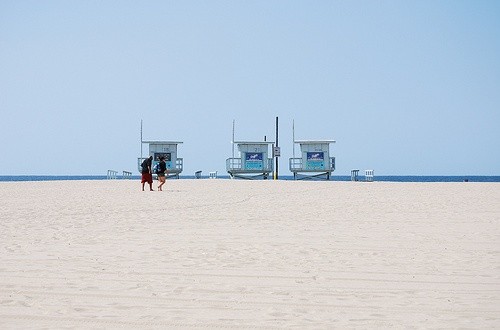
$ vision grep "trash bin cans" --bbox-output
[195,171,202,178]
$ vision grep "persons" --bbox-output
[156,157,168,191]
[140,155,155,191]
